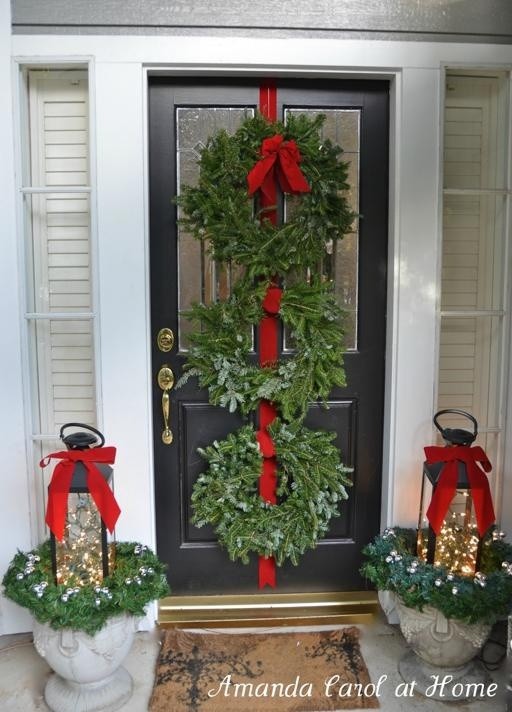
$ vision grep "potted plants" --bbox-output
[356,526,511,702]
[2,540,171,712]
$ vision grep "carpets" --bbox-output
[146,623,380,711]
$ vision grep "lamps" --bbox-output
[416,406,489,577]
[45,420,118,590]
